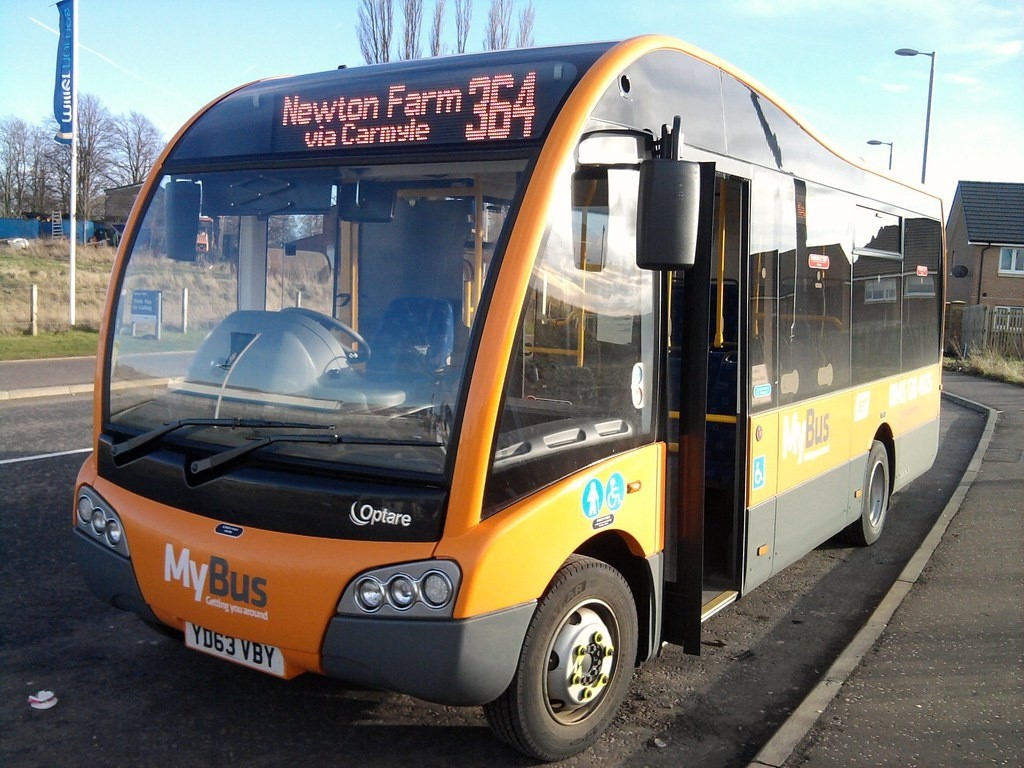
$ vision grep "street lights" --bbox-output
[867,139,894,172]
[891,47,936,184]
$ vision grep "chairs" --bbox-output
[367,295,452,380]
[532,367,598,419]
[727,327,941,405]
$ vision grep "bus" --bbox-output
[67,30,949,763]
